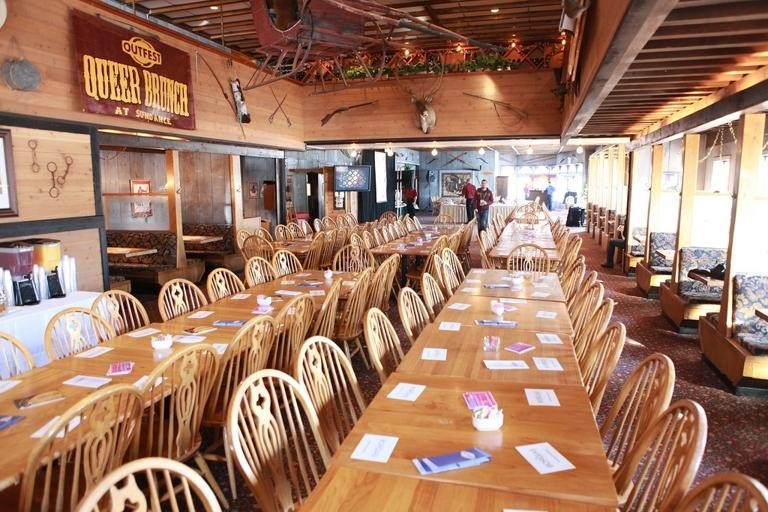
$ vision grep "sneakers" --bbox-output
[601,263,613,268]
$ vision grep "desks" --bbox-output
[2,366,143,491]
[288,463,621,512]
[331,371,621,507]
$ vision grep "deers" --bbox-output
[391,50,446,135]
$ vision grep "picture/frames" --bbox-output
[129,178,154,218]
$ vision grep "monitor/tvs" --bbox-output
[333,164,372,191]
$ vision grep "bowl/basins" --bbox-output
[473,428,502,452]
[490,301,504,313]
[152,331,172,348]
[155,349,172,362]
[324,270,332,279]
[257,293,272,307]
[471,409,504,432]
[483,335,500,352]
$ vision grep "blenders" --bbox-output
[0,240,41,306]
[23,239,65,298]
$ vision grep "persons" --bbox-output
[461,178,477,224]
[472,179,494,237]
[138,186,147,206]
[601,215,627,268]
[402,182,417,221]
[499,197,506,204]
[544,181,555,209]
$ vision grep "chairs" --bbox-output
[19,382,146,510]
[224,367,333,512]
[290,334,368,457]
[599,350,679,510]
[69,454,226,512]
[674,467,768,512]
[2,196,767,402]
[140,342,232,512]
[580,320,627,420]
[613,397,710,512]
[199,313,278,501]
